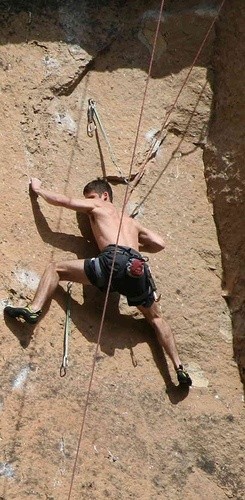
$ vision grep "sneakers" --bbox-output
[175,366,195,386]
[5,304,43,324]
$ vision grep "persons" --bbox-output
[3,174,197,383]
